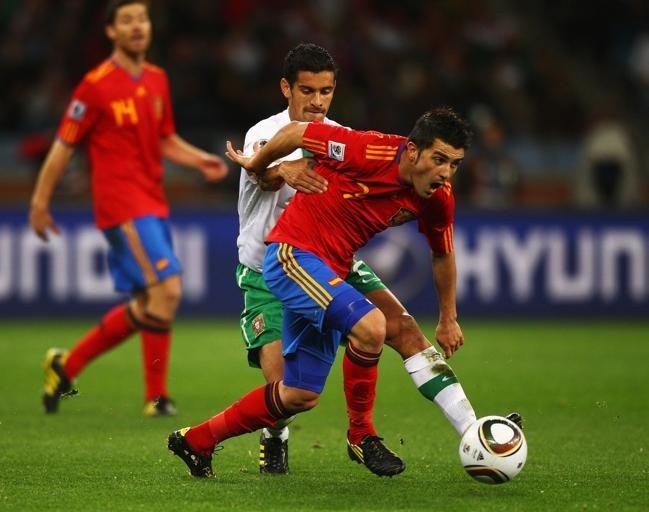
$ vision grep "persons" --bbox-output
[27,0,231,420]
[1,0,649,215]
[164,105,474,483]
[229,42,527,477]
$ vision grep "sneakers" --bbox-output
[166,428,215,480]
[256,424,290,477]
[39,347,73,416]
[504,410,522,429]
[342,427,406,478]
[141,399,178,420]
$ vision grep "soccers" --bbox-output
[459,416,527,483]
